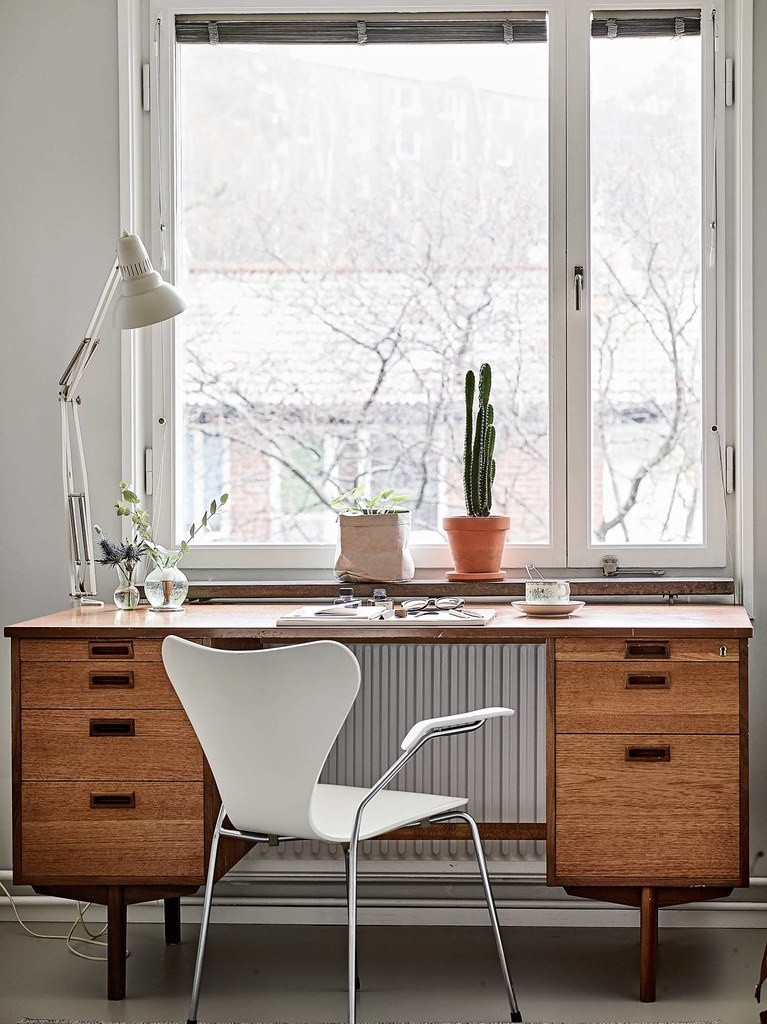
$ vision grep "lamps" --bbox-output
[58,230,187,610]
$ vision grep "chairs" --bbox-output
[161,635,521,1024]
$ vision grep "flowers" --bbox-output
[94,525,148,606]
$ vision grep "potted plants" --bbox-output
[332,486,415,581]
[443,364,510,581]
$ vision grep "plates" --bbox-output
[512,601,586,613]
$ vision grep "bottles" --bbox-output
[334,588,362,607]
[368,589,393,609]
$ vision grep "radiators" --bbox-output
[251,643,548,861]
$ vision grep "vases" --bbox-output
[144,552,189,612]
[114,563,140,610]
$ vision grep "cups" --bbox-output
[525,580,571,603]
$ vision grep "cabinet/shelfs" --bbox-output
[3,602,753,1002]
